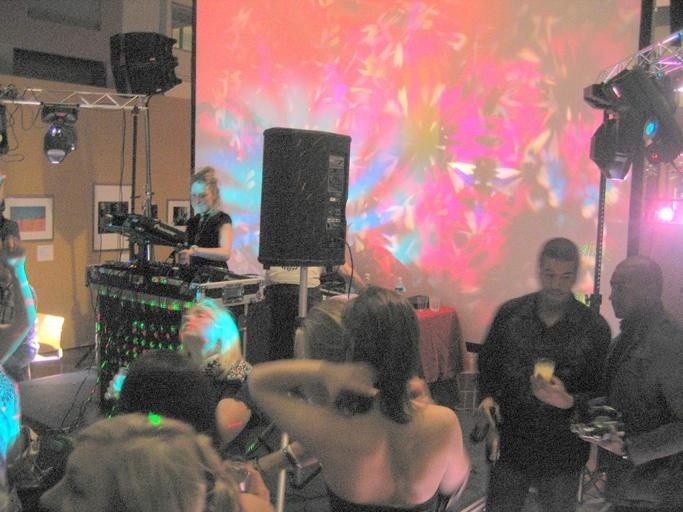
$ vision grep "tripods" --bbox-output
[75,111,139,366]
[246,267,325,512]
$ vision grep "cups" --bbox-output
[534,357,556,384]
[416,291,442,312]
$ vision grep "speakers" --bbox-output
[257,127,352,270]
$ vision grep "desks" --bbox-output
[414,306,461,406]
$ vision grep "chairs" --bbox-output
[23,314,66,381]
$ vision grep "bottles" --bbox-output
[395,277,406,294]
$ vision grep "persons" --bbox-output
[172,165,232,269]
[0,233,37,511]
[108,346,277,512]
[474,236,612,511]
[260,254,364,312]
[102,294,257,451]
[37,413,243,511]
[247,284,469,511]
[565,252,682,511]
[0,194,37,384]
